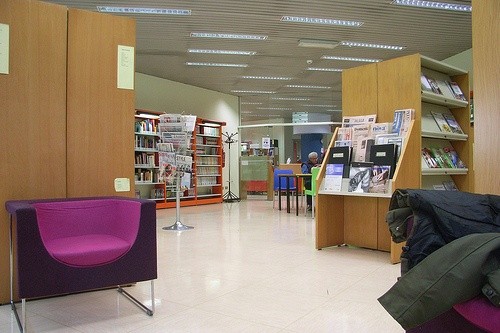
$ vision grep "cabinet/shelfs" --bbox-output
[134,108,227,209]
[316,53,473,264]
[0,0,135,304]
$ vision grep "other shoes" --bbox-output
[307,205,312,211]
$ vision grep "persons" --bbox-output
[301,152,322,211]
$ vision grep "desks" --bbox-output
[280,164,304,197]
[278,174,312,215]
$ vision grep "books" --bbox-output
[421,75,468,192]
[324,109,416,194]
[134,113,220,198]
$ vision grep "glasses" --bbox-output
[310,157,318,160]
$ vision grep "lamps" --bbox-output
[298,39,339,49]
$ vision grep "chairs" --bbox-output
[301,167,320,218]
[5,196,157,333]
[401,215,500,333]
[273,169,296,209]
